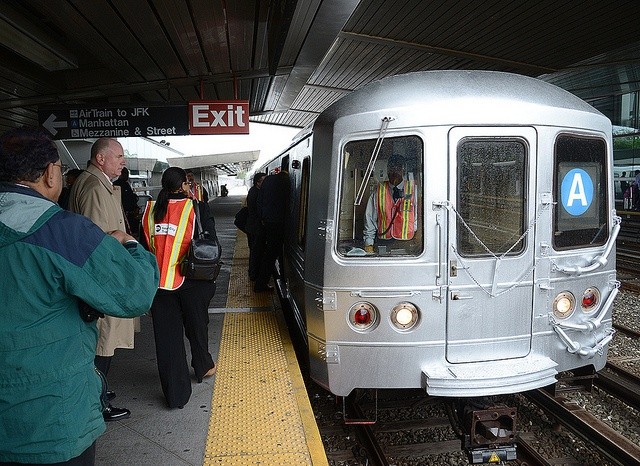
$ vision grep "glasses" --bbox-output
[42,161,68,176]
[185,181,191,185]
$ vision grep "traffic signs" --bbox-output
[39,106,189,139]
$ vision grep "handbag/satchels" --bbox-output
[234,186,261,235]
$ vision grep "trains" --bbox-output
[247,70,622,424]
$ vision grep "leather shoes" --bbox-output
[101,403,131,421]
[254,284,275,293]
[104,390,116,400]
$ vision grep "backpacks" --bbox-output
[179,199,224,280]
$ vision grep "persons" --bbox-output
[254,163,297,292]
[69,139,141,421]
[65,169,80,184]
[363,155,419,252]
[1,135,160,465]
[621,169,640,212]
[139,167,222,409]
[246,173,268,282]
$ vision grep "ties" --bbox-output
[393,187,400,201]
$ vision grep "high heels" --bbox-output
[197,363,217,382]
